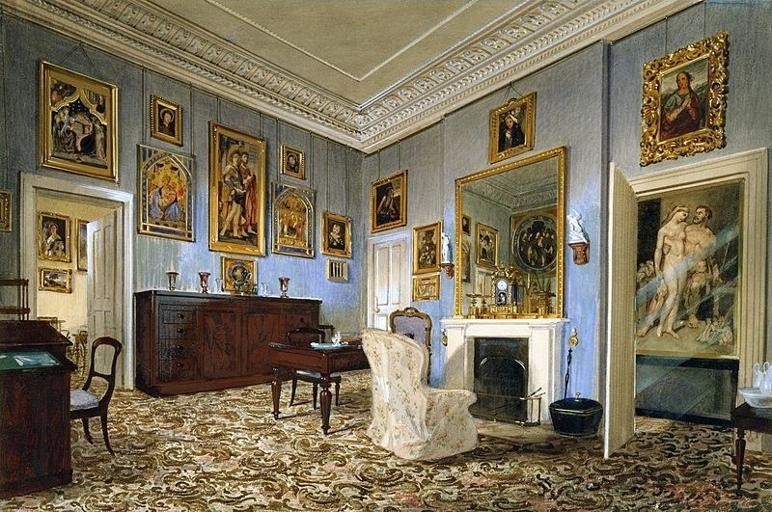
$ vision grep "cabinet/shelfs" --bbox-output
[1,278,31,320]
[132,289,323,399]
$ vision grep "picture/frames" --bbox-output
[486,90,538,166]
[319,209,353,260]
[364,170,443,303]
[631,177,747,362]
[150,96,187,146]
[206,118,271,260]
[135,141,198,247]
[325,257,350,284]
[278,143,308,181]
[461,202,556,282]
[639,30,730,169]
[1,60,121,294]
[219,254,261,294]
[271,178,319,261]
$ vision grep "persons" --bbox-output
[566,209,587,243]
[685,205,716,326]
[654,204,687,340]
[660,71,704,142]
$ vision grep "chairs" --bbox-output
[389,306,432,384]
[67,334,124,459]
[288,325,342,409]
[361,328,480,463]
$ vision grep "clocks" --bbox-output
[487,262,519,314]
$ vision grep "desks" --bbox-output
[1,319,79,500]
[729,401,772,493]
[267,338,370,435]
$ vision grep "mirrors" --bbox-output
[453,145,568,322]
